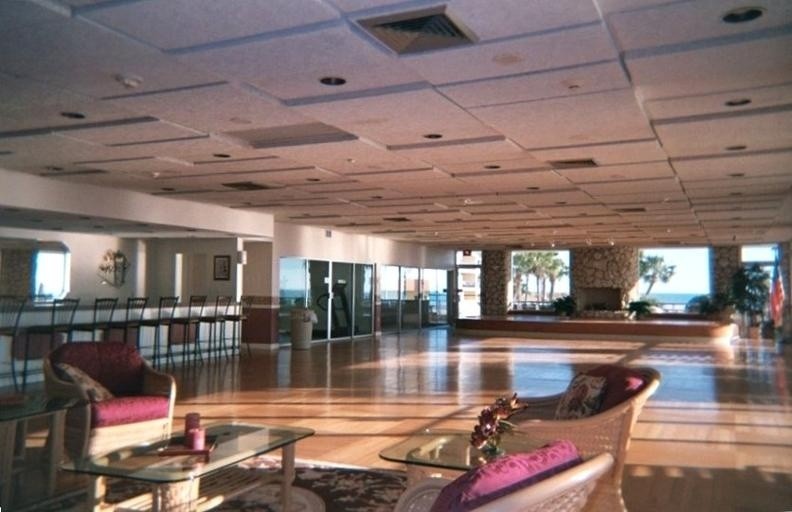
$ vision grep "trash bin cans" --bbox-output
[289,307,314,352]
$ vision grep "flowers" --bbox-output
[471,391,530,466]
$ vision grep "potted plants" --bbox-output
[553,296,577,316]
[629,300,651,320]
[731,263,770,339]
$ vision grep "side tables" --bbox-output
[1,391,81,506]
[379,429,539,479]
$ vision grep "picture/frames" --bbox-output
[213,255,231,280]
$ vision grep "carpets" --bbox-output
[15,454,409,511]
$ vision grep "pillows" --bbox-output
[429,440,582,512]
[552,370,645,421]
[55,362,114,403]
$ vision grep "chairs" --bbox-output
[172,295,208,368]
[505,365,661,511]
[110,297,149,352]
[22,298,80,392]
[201,294,233,362]
[393,437,614,511]
[224,295,255,360]
[44,341,177,463]
[142,296,179,371]
[0,294,28,393]
[73,297,118,343]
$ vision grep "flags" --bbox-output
[769,253,785,328]
